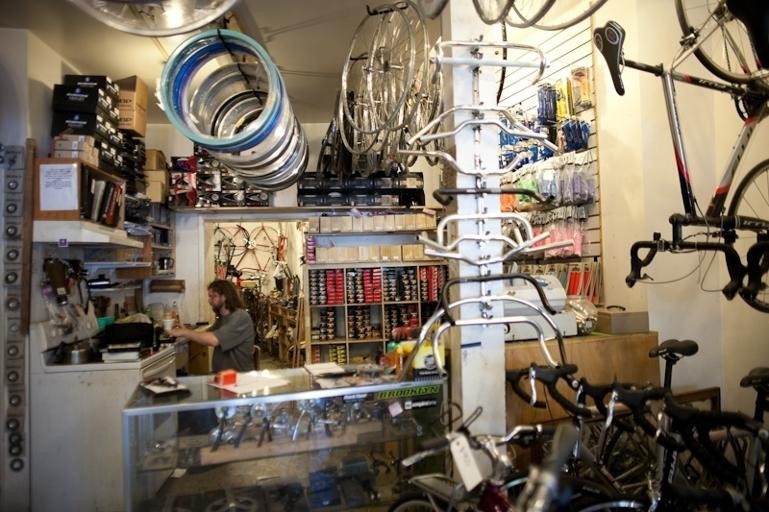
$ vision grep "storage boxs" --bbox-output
[49,73,271,209]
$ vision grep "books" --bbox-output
[304,362,345,376]
[100,341,141,364]
[136,375,191,398]
[80,167,124,229]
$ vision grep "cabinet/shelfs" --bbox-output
[305,259,452,364]
[122,363,452,511]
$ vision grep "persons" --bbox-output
[165,278,257,438]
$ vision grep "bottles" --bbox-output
[164,301,180,328]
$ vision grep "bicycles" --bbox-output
[592,3,765,314]
[391,340,769,512]
